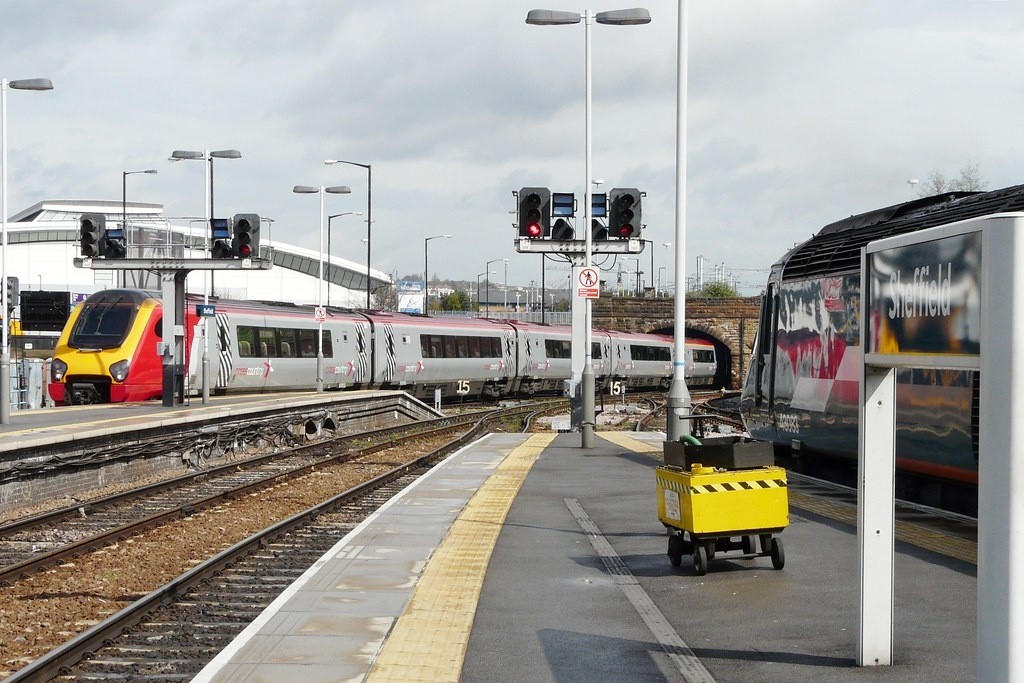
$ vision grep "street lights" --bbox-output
[120,168,159,261]
[425,234,452,315]
[658,266,666,294]
[477,271,497,318]
[522,7,654,450]
[660,243,671,294]
[290,184,353,396]
[327,212,364,308]
[1,76,55,427]
[170,146,245,295]
[322,159,373,310]
[486,258,510,320]
[592,178,606,193]
[621,256,640,298]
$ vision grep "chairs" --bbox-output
[240,341,251,355]
[426,346,436,357]
[554,349,559,357]
[254,342,268,355]
[275,342,290,355]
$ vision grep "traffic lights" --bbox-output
[608,188,640,239]
[78,213,107,257]
[518,186,551,238]
[105,241,124,258]
[214,240,232,259]
[233,212,260,259]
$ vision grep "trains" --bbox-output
[734,182,1023,519]
[43,286,718,397]
